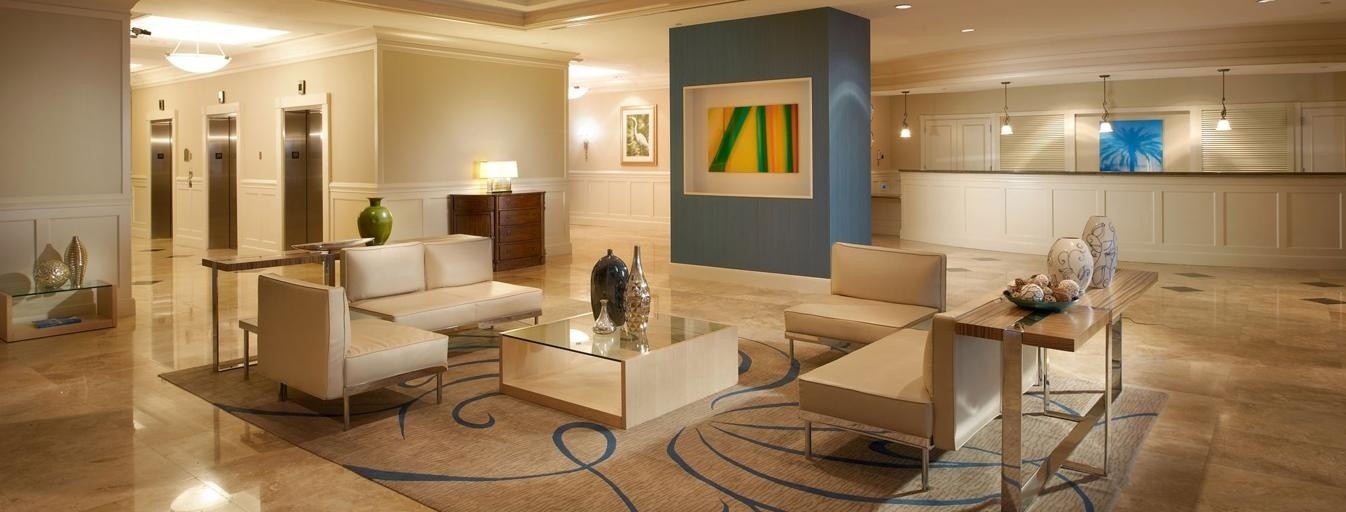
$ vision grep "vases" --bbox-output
[357,197,394,246]
[1083,216,1118,288]
[623,246,650,335]
[590,250,629,327]
[1047,236,1094,293]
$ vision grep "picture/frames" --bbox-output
[620,104,658,167]
[683,77,814,200]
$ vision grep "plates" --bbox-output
[1003,288,1080,311]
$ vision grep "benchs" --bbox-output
[339,236,543,335]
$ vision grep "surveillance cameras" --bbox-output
[132,27,151,36]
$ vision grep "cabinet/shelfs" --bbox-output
[0,281,119,344]
[448,190,546,273]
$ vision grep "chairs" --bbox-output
[258,272,450,430]
[800,294,1044,491]
[783,241,947,367]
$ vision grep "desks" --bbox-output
[201,249,329,374]
[953,266,1159,511]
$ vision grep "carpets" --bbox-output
[159,335,1170,512]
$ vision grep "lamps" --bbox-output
[165,23,231,74]
[1000,81,1013,135]
[479,160,518,193]
[1216,68,1231,131]
[900,91,911,139]
[1098,75,1114,132]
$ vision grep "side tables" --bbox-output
[238,317,258,380]
